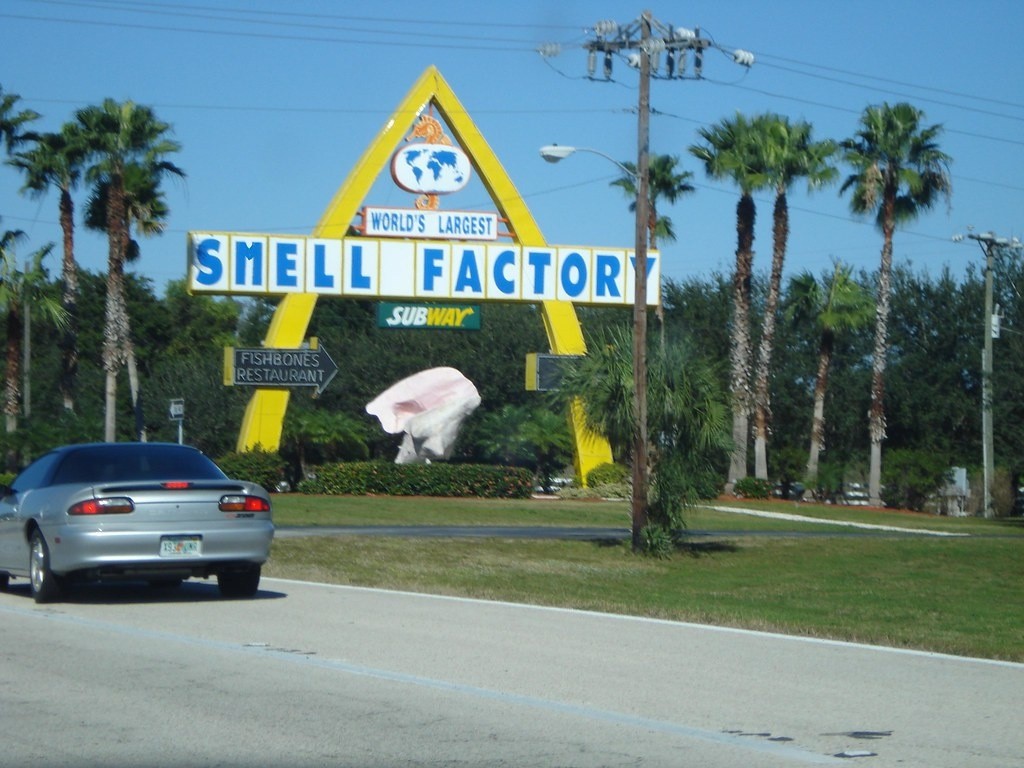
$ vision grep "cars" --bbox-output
[0,441,275,605]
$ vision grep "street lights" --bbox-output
[543,144,650,556]
